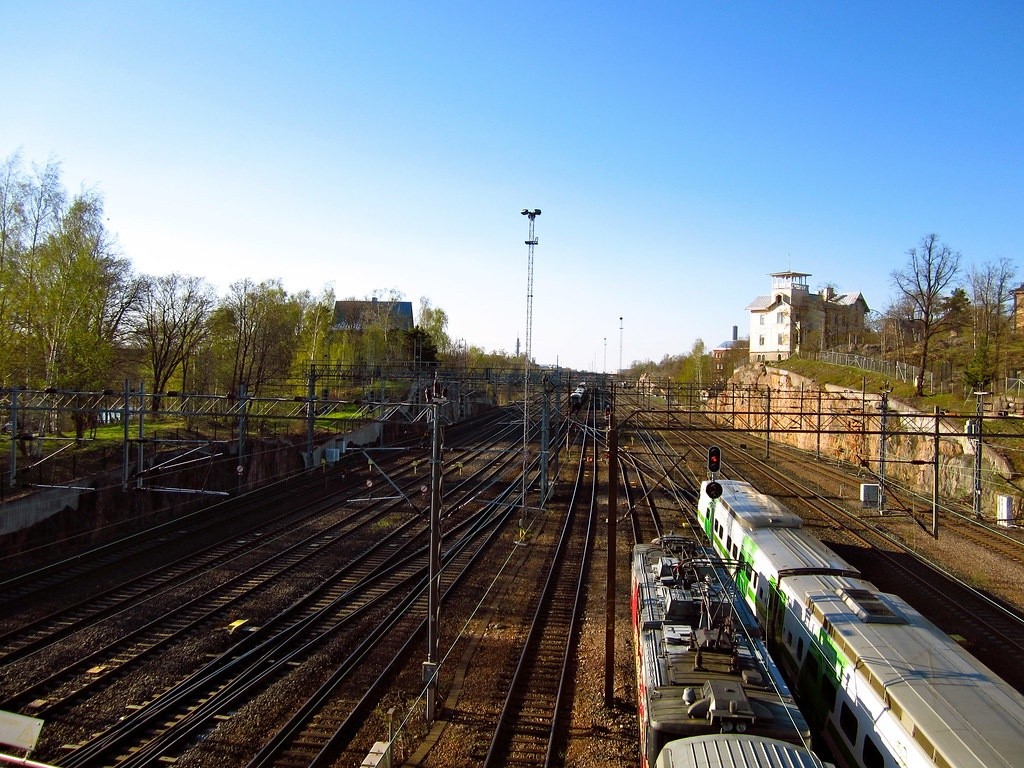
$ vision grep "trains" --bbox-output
[630,534,833,766]
[569,380,588,407]
[697,481,1024,768]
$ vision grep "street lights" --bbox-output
[877,380,895,515]
[419,380,445,705]
[520,207,544,503]
[972,381,989,521]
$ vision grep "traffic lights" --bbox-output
[709,446,721,474]
[706,482,723,499]
